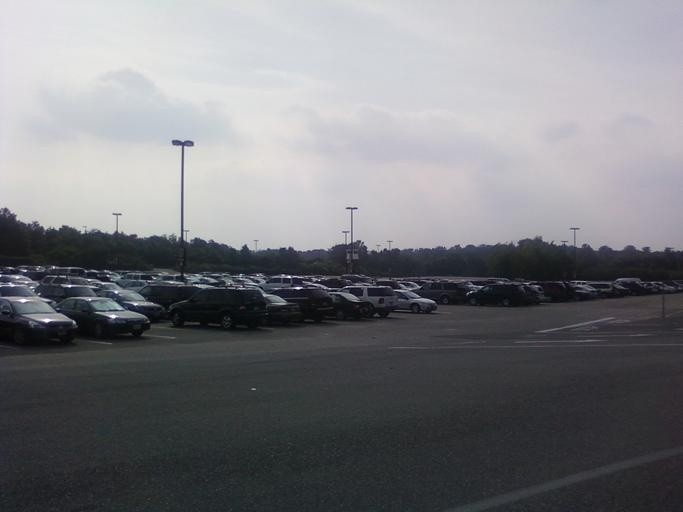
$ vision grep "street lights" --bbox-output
[253,239,259,250]
[83,226,89,234]
[183,229,189,242]
[377,245,382,252]
[341,230,349,245]
[171,140,195,281]
[113,212,122,233]
[560,240,568,246]
[344,206,359,272]
[387,241,392,251]
[569,227,580,249]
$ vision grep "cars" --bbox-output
[439,276,683,310]
